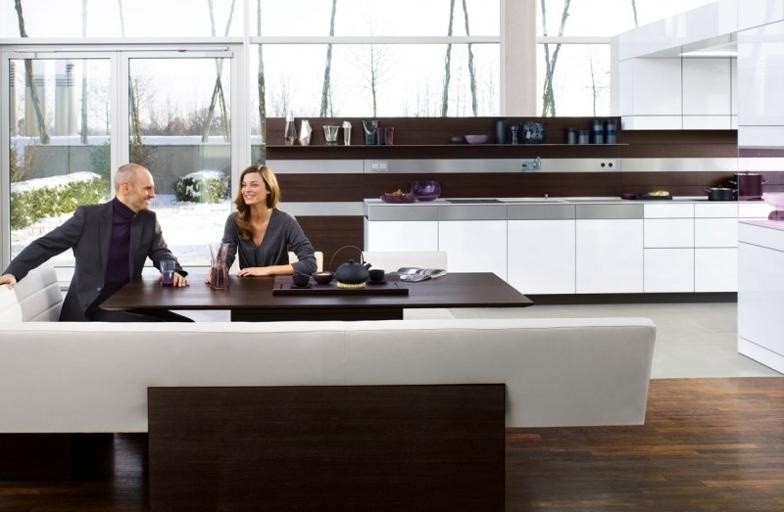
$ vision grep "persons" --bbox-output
[203,163,318,285]
[0,162,197,322]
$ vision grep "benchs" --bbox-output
[0,261,656,512]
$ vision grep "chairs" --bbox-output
[287,252,324,272]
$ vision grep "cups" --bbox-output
[159,259,177,287]
[369,270,385,282]
[208,243,231,291]
[494,120,508,144]
[321,119,394,145]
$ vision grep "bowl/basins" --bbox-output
[293,274,309,287]
[410,180,442,202]
[464,134,489,145]
[311,271,334,284]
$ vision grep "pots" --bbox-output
[704,184,739,202]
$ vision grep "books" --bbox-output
[389,266,447,282]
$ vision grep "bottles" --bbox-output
[511,125,520,145]
[567,119,617,144]
[283,108,312,146]
[616,190,673,200]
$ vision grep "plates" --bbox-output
[380,193,416,203]
[368,279,388,285]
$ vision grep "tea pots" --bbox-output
[330,245,372,284]
